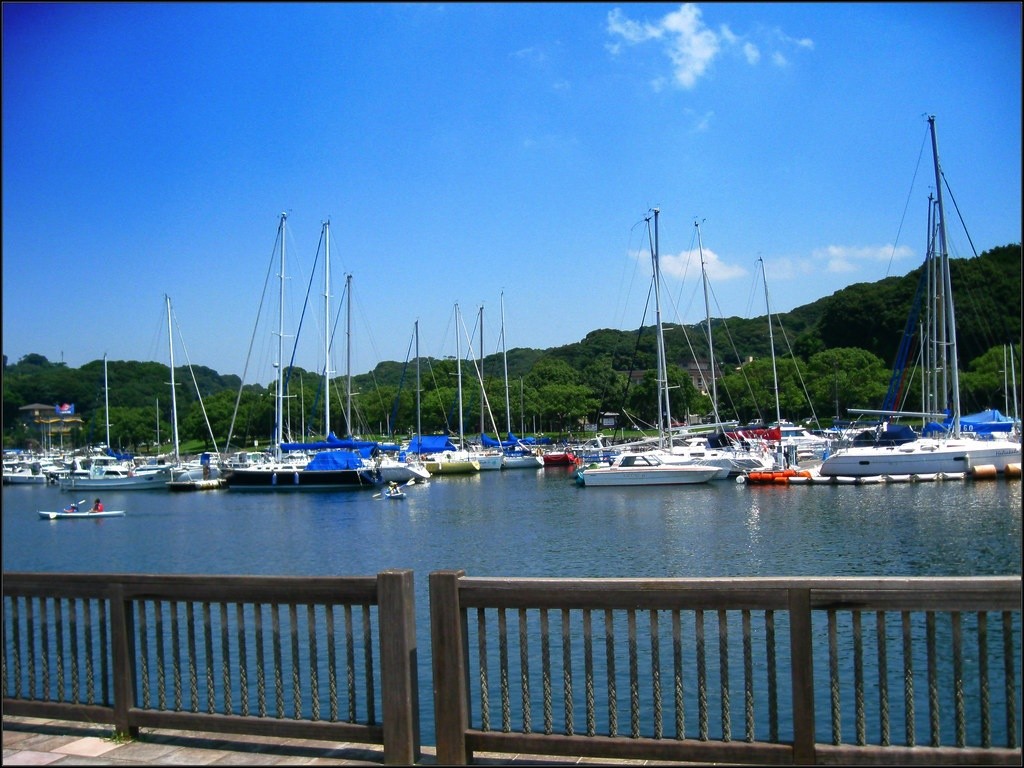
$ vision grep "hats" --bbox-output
[95,498,100,503]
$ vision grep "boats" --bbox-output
[37,510,126,518]
[385,492,408,498]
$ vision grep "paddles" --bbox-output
[49,499,85,518]
[372,477,416,498]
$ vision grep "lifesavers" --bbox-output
[756,443,768,452]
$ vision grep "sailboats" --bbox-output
[3,114,1024,492]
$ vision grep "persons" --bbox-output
[89,499,103,513]
[64,503,78,513]
[387,481,401,496]
